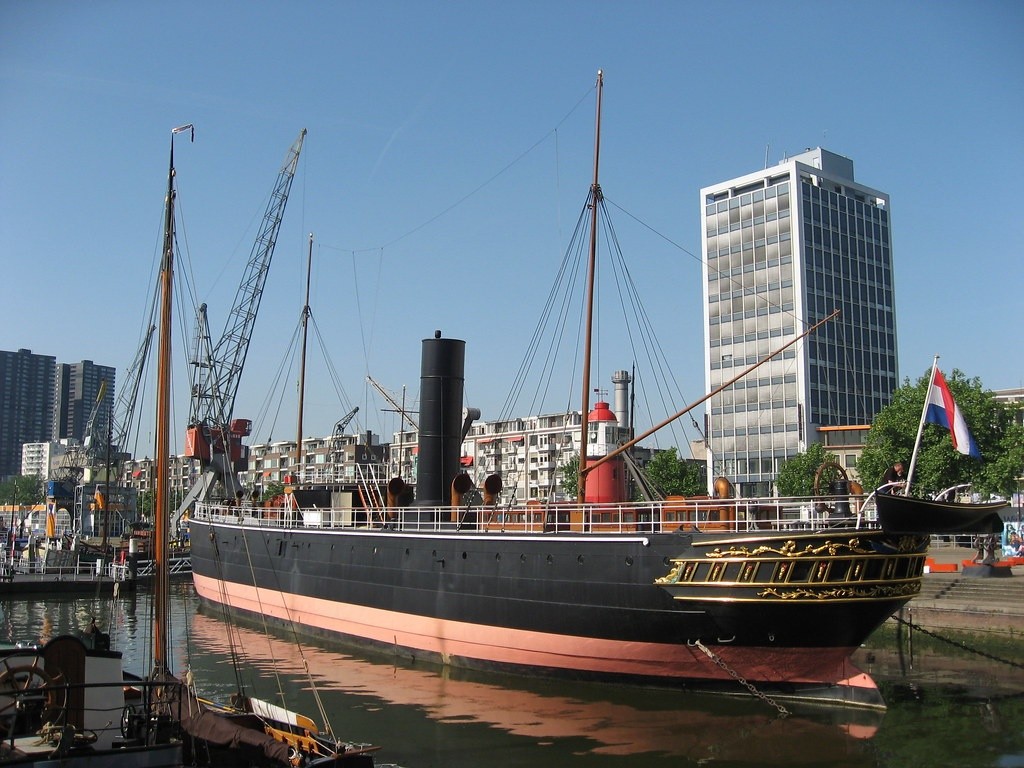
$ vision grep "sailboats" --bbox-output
[186,65,1012,710]
[0,124,401,768]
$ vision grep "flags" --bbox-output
[924,367,982,459]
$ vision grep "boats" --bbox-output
[18,532,80,573]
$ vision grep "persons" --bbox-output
[875,462,906,530]
[1010,531,1020,551]
